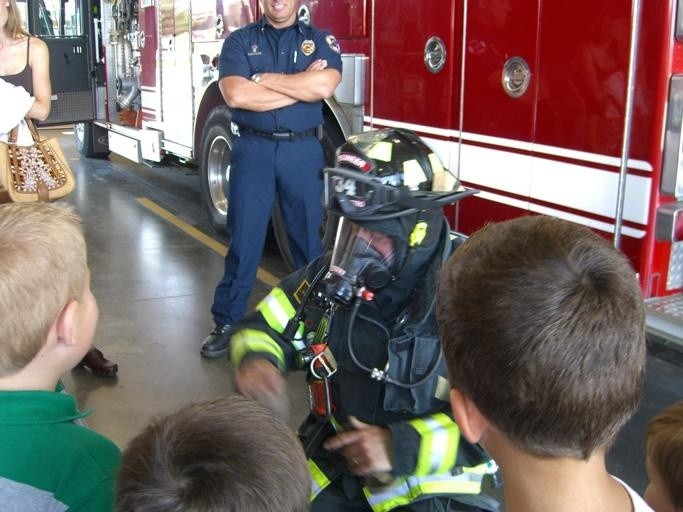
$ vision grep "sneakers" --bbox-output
[198,320,243,360]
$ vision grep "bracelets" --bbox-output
[250,72,261,83]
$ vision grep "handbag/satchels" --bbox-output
[0,112,77,204]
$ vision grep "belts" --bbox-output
[236,117,317,143]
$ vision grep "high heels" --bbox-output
[74,346,119,380]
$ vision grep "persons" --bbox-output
[642,400,682,511]
[232,123,500,511]
[0,198,125,512]
[1,1,51,206]
[195,3,344,358]
[115,393,313,511]
[437,212,657,511]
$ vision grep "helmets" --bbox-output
[329,127,446,219]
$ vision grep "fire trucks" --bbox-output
[26,0,683,353]
